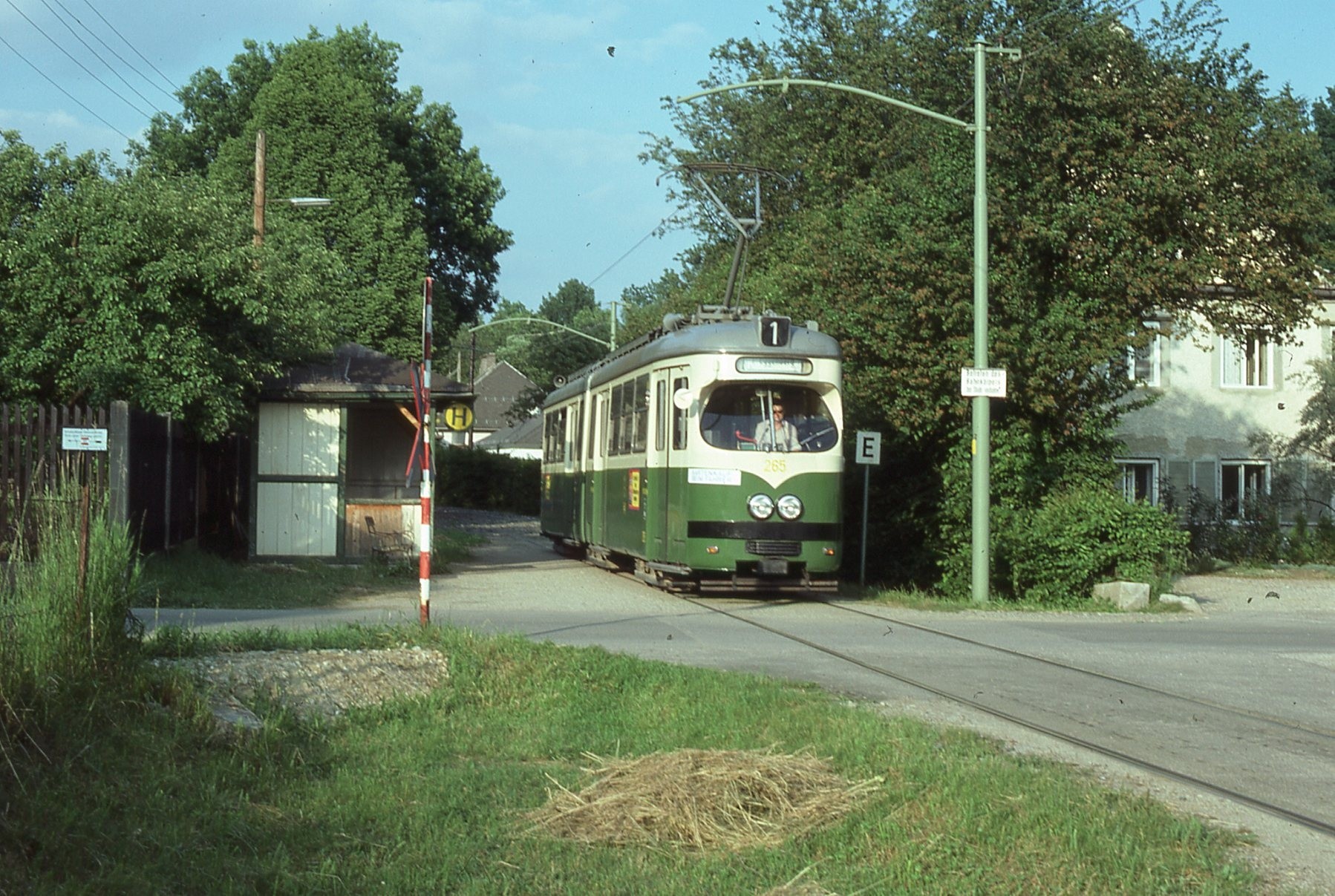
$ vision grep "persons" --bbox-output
[755,401,802,454]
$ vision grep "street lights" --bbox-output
[227,197,332,560]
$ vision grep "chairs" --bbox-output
[365,516,416,572]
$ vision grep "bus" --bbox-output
[538,161,847,595]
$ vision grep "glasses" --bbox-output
[773,409,785,414]
